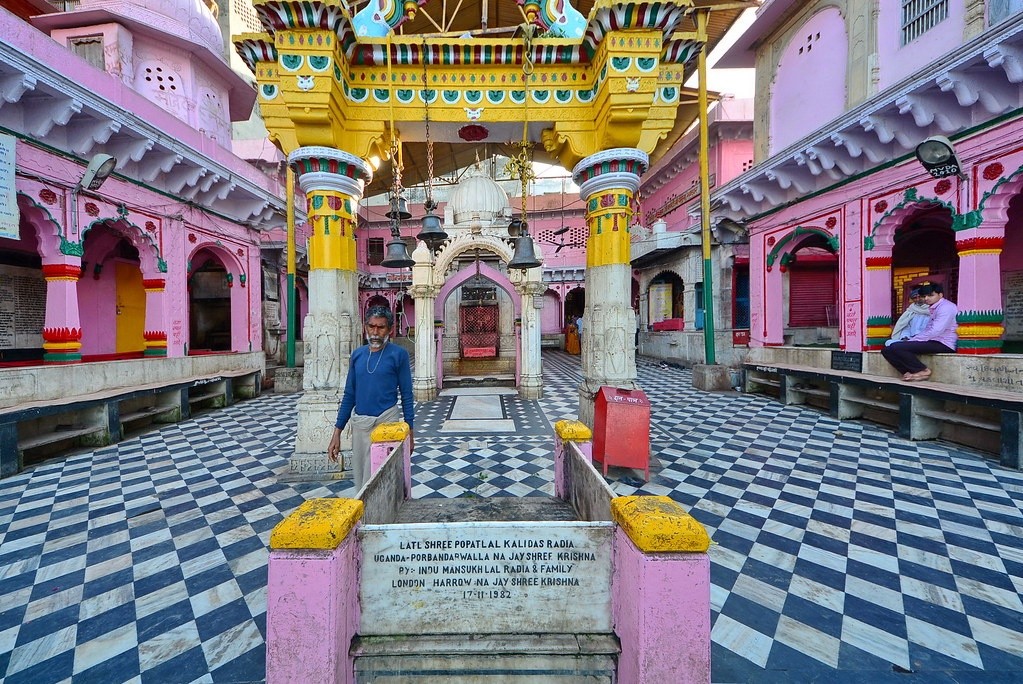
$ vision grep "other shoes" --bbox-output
[903,372,911,381]
[909,368,931,380]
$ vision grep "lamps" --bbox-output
[715,219,750,244]
[508,218,530,236]
[915,135,970,182]
[71,153,118,234]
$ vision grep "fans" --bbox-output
[546,178,583,253]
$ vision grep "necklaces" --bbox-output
[366,343,386,373]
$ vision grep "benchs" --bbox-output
[0,369,262,480]
[741,362,1023,470]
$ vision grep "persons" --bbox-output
[880,282,958,383]
[566,313,584,355]
[328,305,415,496]
